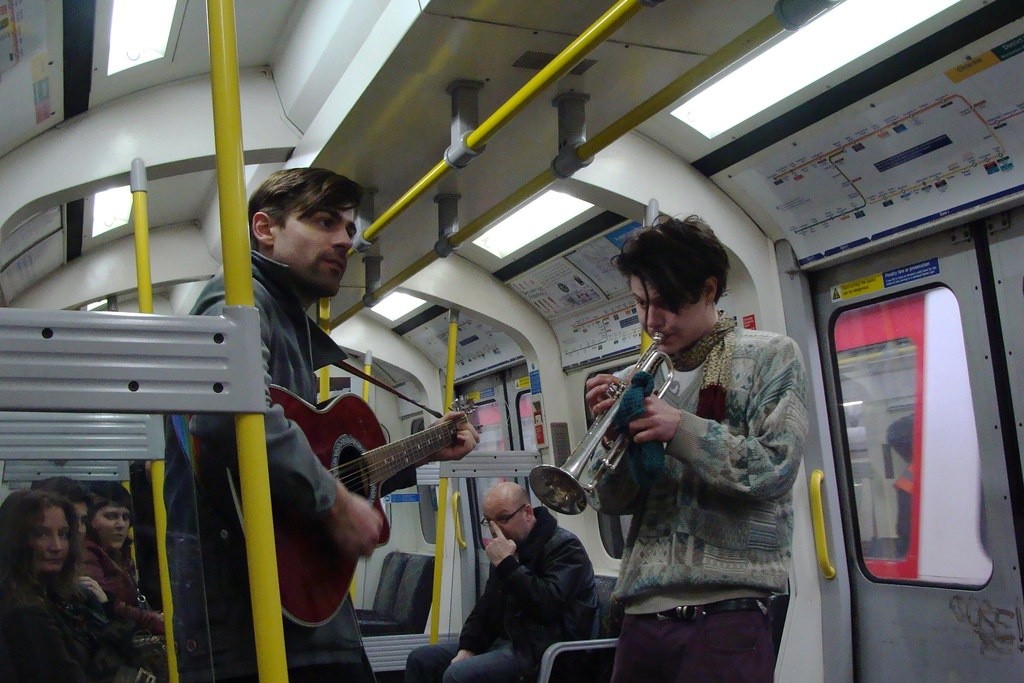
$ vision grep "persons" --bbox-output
[0,477,170,682]
[163,167,479,683]
[404,482,600,683]
[584,212,810,682]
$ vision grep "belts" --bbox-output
[627,597,773,623]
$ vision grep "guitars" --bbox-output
[185,382,485,630]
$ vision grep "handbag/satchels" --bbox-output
[125,628,179,677]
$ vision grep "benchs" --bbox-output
[355,551,626,683]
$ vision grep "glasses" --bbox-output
[479,504,527,529]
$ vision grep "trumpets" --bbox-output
[528,330,675,517]
